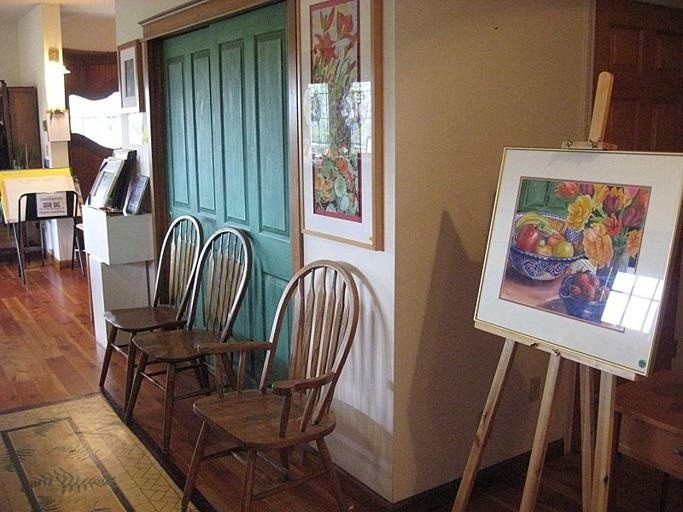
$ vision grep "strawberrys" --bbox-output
[568,271,609,302]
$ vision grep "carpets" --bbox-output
[1,390,218,511]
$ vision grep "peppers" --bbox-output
[516,222,574,258]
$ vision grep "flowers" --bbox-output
[553,181,651,270]
[312,6,358,124]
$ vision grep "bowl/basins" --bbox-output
[510,212,586,280]
[559,273,621,323]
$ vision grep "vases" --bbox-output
[593,240,625,296]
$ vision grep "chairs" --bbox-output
[179,260,360,511]
[98,215,208,411]
[124,228,254,455]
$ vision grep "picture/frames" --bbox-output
[300,0,383,252]
[117,39,144,116]
[472,146,683,377]
[85,147,150,218]
